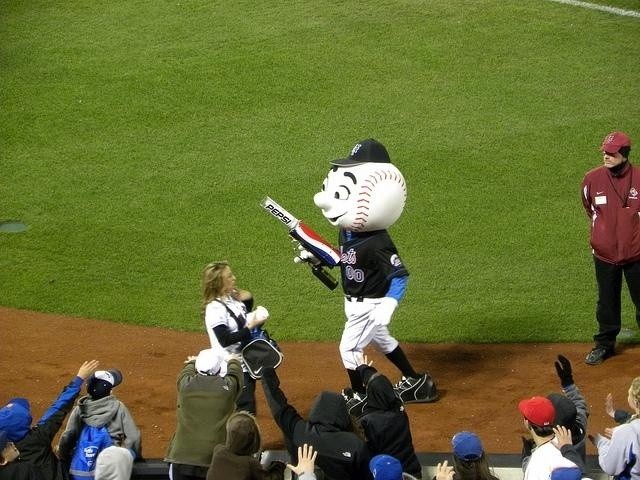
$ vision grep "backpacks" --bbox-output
[69,424,112,480]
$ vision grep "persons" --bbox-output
[203,260,287,420]
[292,137,438,414]
[165,347,422,480]
[580,133,639,367]
[434,431,499,480]
[519,353,640,480]
[0,359,142,480]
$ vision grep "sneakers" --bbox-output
[585,346,615,365]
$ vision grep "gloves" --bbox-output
[520,436,534,459]
[554,355,574,387]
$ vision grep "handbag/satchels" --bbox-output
[241,328,284,380]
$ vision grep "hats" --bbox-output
[548,393,577,429]
[599,132,631,153]
[369,454,402,480]
[518,396,556,426]
[86,368,123,394]
[551,467,583,480]
[0,431,8,449]
[451,431,482,460]
[195,348,222,376]
[0,398,32,441]
